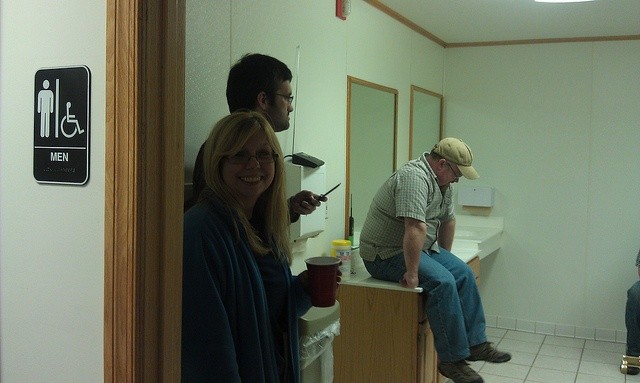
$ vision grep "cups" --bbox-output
[351,244,360,275]
[305,256,341,308]
[330,239,353,278]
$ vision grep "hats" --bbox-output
[432,137,481,180]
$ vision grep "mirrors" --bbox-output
[344,75,397,250]
[410,84,444,160]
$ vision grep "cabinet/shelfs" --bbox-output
[335,251,480,383]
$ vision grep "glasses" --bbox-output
[224,150,279,163]
[446,162,461,180]
[276,92,295,103]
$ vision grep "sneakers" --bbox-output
[438,359,484,383]
[466,342,511,363]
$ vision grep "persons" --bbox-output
[359,137,512,383]
[625,249,640,376]
[193,54,328,224]
[181,112,342,383]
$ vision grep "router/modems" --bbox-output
[293,153,325,168]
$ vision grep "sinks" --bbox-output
[452,229,475,237]
